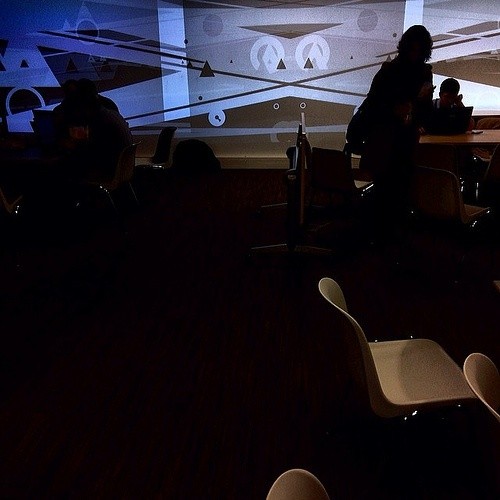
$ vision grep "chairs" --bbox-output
[135,127,177,204]
[410,115,500,255]
[268,468,331,500]
[463,353,500,424]
[316,275,480,418]
[88,138,144,208]
[303,135,352,216]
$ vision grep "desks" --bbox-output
[418,129,500,181]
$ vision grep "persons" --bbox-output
[52,80,132,181]
[347,25,465,231]
